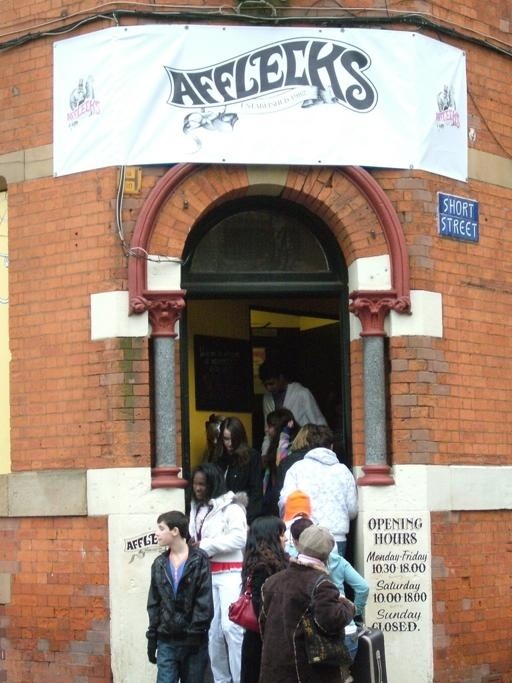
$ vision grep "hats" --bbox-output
[298,523,335,561]
[283,489,310,520]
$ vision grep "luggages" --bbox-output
[350,621,387,682]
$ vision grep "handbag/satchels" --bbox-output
[229,561,272,632]
[301,574,354,664]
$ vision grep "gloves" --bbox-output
[183,636,201,657]
[148,638,157,663]
